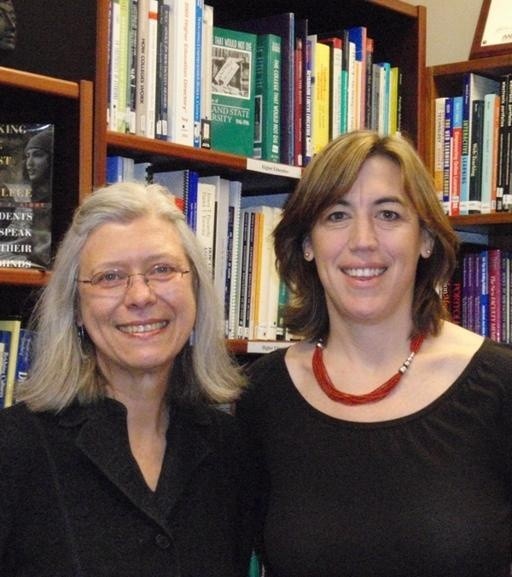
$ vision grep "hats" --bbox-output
[25,130,52,152]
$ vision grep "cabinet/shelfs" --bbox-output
[0,0,427,410]
[428,58,508,347]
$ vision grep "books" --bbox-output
[108,0,213,149]
[255,15,402,166]
[154,171,197,229]
[2,123,54,271]
[108,158,151,187]
[212,27,255,154]
[192,175,309,342]
[0,318,35,406]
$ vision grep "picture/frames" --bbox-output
[470,0,508,58]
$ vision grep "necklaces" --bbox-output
[313,322,425,405]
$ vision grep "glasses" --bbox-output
[74,263,189,291]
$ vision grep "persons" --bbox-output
[435,72,512,217]
[234,131,512,576]
[438,249,510,346]
[0,179,249,575]
[25,128,53,269]
